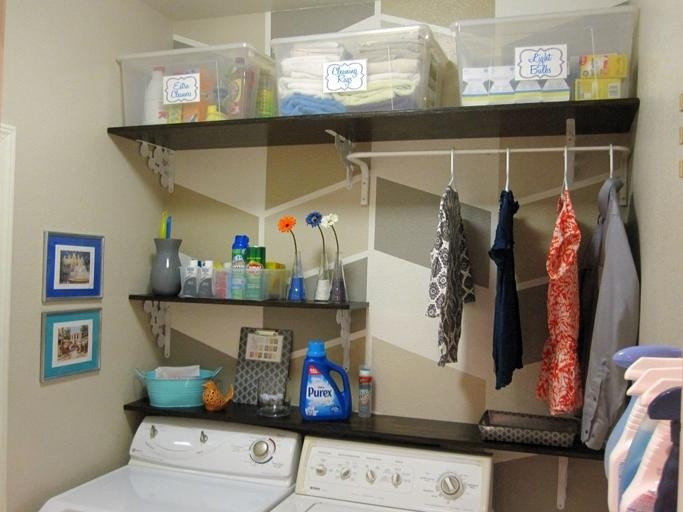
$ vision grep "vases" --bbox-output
[286,249,307,300]
[330,250,347,302]
[314,252,331,301]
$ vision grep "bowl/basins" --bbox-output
[132,366,223,408]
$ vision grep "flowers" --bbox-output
[307,207,327,276]
[320,211,342,272]
[276,211,301,278]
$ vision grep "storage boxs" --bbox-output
[453,1,641,107]
[269,21,448,115]
[173,261,290,303]
[110,40,276,126]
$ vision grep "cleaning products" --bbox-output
[299,338,352,423]
[182,234,266,301]
[141,55,278,128]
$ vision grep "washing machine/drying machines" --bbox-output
[266,435,494,512]
[38,414,303,512]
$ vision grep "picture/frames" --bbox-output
[40,228,105,304]
[37,306,102,385]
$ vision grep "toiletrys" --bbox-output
[358,365,373,420]
[141,57,274,125]
[181,235,265,299]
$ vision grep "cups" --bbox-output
[257,378,290,418]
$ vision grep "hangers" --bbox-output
[607,144,616,182]
[446,148,457,190]
[501,148,511,191]
[558,147,569,197]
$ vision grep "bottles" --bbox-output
[357,368,372,418]
[143,57,273,125]
[229,235,267,303]
